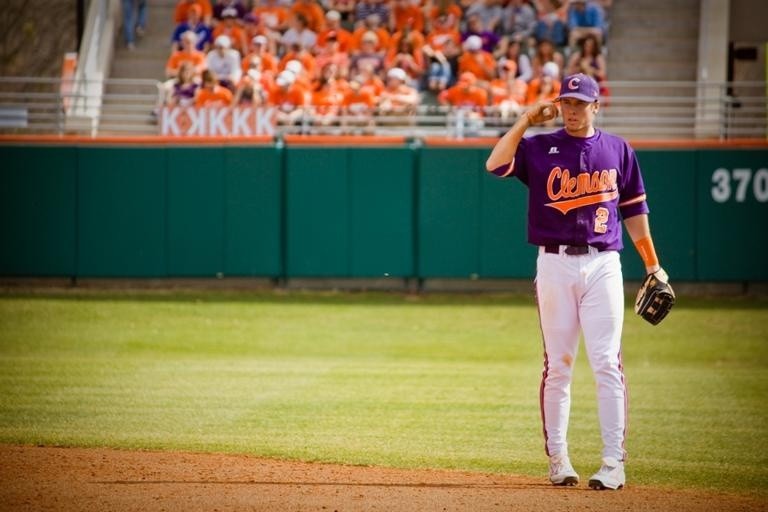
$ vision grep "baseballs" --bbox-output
[543,107,551,116]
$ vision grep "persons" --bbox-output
[485,73,673,488]
[123,1,148,49]
[148,1,613,138]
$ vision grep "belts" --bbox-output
[545,245,604,254]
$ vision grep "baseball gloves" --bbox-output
[634,273,674,325]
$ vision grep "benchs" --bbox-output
[97,1,696,141]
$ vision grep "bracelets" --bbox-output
[526,111,535,126]
[633,238,659,266]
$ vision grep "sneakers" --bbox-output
[549,451,579,486]
[588,462,625,490]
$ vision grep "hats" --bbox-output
[554,72,599,102]
